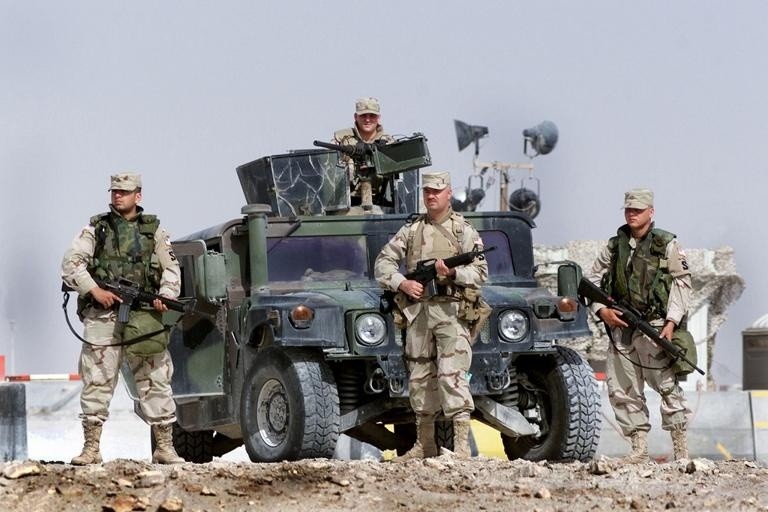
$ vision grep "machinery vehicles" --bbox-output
[142,134,604,466]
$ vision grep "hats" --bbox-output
[419,171,451,191]
[620,189,654,210]
[354,98,381,116]
[108,173,143,192]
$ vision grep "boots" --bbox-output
[608,431,651,468]
[71,423,103,465]
[152,425,186,464]
[439,419,471,462]
[390,414,439,463]
[670,429,689,473]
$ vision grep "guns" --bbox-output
[62,276,216,320]
[577,277,707,376]
[377,245,497,317]
[313,141,376,164]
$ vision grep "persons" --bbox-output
[374,169,489,463]
[584,188,692,465]
[61,172,187,465]
[328,96,399,207]
